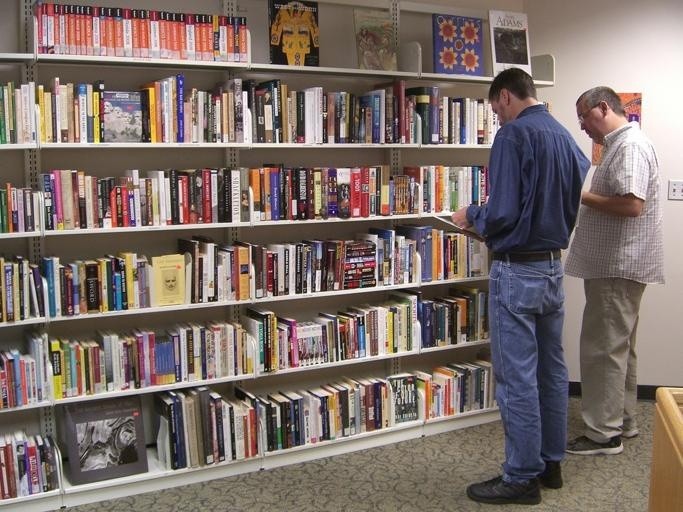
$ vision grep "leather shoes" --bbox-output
[538,459,563,490]
[467,474,542,506]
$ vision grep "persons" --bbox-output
[563,86,665,458]
[451,66,591,507]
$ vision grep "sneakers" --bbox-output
[565,433,625,455]
[621,426,640,438]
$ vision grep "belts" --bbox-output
[492,251,564,263]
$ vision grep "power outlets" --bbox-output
[667,179,683,201]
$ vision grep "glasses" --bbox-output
[578,100,604,124]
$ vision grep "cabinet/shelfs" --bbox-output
[0,0,555,510]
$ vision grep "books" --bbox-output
[487,8,532,77]
[0,76,501,145]
[354,8,397,73]
[0,289,489,410]
[0,224,491,326]
[431,215,485,243]
[32,1,248,63]
[0,353,496,500]
[431,12,483,77]
[265,0,320,68]
[0,164,489,232]
